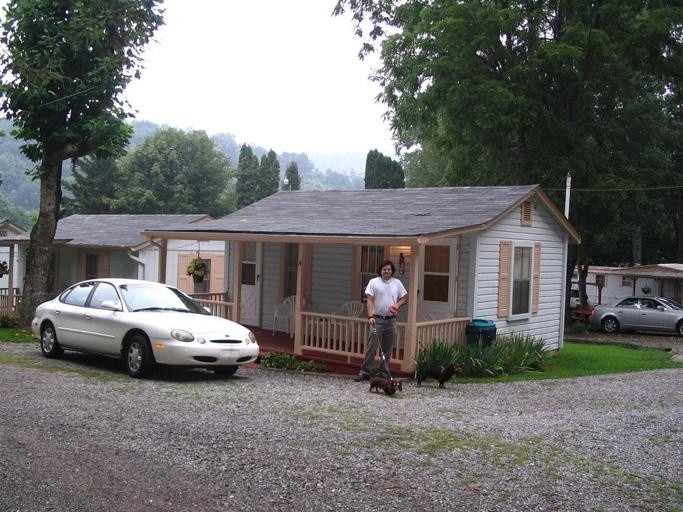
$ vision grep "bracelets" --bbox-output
[367,315,373,320]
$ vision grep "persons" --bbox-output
[353,259,408,381]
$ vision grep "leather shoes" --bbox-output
[355,373,370,381]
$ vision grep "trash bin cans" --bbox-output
[465,319,497,345]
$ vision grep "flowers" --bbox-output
[186,258,208,277]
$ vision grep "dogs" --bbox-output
[369,375,403,395]
[414,363,462,389]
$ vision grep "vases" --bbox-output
[192,273,204,282]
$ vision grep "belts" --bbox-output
[374,315,396,319]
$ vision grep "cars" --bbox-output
[30,277,260,380]
[590,296,683,337]
[569,289,580,308]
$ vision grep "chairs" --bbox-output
[271,295,295,339]
[332,300,364,345]
